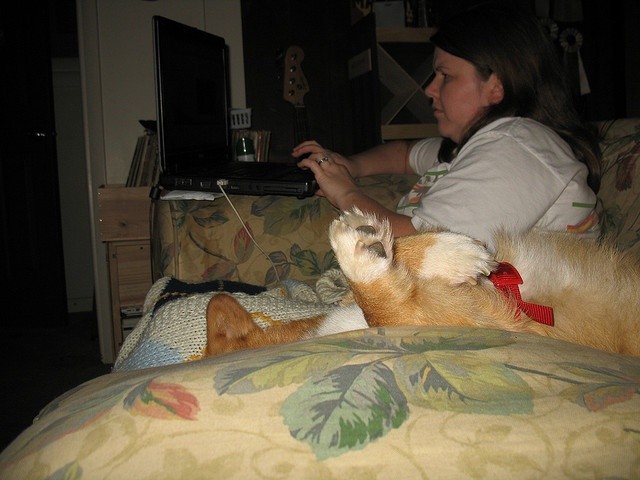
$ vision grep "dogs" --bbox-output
[204,207,640,358]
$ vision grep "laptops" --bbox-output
[151,15,317,196]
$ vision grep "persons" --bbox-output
[290,0,602,240]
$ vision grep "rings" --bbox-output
[317,156,328,165]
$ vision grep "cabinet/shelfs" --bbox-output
[375,24,441,140]
[108,241,152,360]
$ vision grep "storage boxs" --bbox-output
[96,184,155,238]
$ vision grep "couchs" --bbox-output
[1,114,639,478]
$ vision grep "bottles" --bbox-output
[235,127,256,161]
